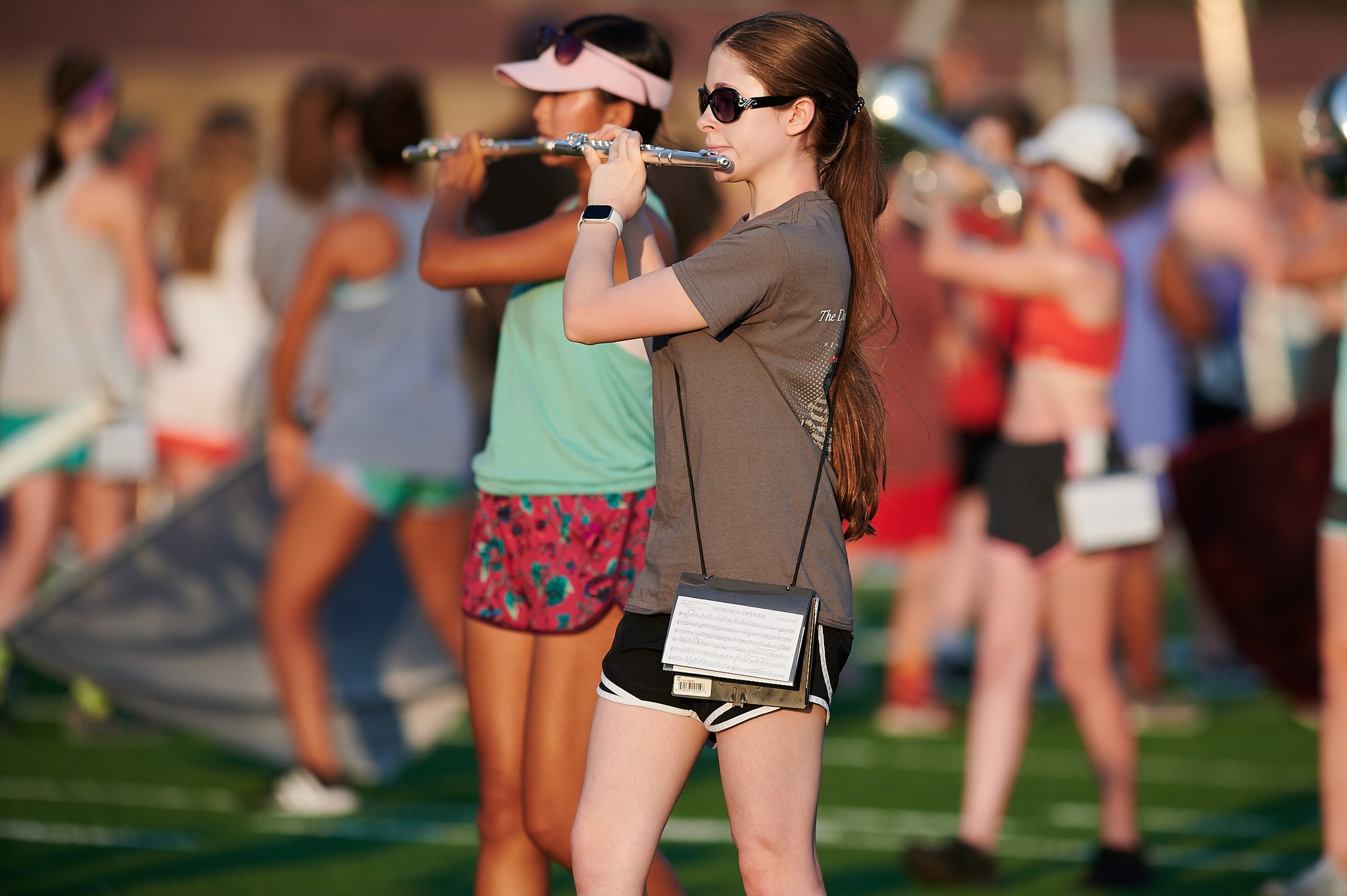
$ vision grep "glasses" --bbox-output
[523,25,651,105]
[696,87,797,124]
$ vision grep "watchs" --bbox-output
[577,203,624,238]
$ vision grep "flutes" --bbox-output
[406,134,580,165]
[543,130,732,173]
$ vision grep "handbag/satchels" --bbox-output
[1051,463,1163,559]
[661,569,824,713]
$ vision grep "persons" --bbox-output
[1258,69,1347,895]
[563,12,899,895]
[1,47,176,726]
[152,102,268,494]
[418,13,674,894]
[854,56,1280,895]
[251,69,365,449]
[265,70,472,817]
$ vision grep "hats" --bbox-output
[1017,102,1140,192]
[492,35,675,115]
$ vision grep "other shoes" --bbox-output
[246,765,360,819]
[874,698,962,742]
[1122,698,1206,737]
[901,837,999,886]
[1078,843,1146,894]
